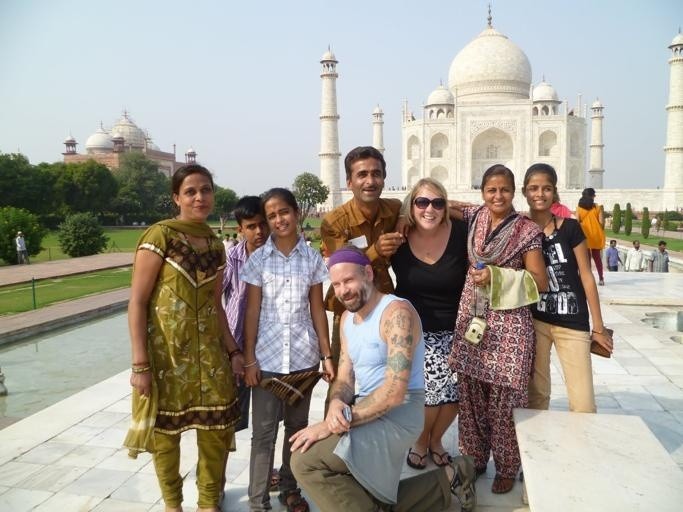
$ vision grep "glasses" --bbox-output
[415,197,445,209]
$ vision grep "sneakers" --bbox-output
[449,455,476,512]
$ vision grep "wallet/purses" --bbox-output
[590,329,613,358]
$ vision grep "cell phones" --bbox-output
[341,407,352,423]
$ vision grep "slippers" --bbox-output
[492,473,514,493]
[407,448,449,469]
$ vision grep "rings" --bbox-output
[302,437,308,442]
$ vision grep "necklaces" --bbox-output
[183,233,212,272]
[533,217,552,229]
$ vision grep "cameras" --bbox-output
[464,317,488,344]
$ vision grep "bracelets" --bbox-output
[321,356,333,359]
[398,215,405,218]
[228,348,241,361]
[592,328,605,334]
[243,360,258,368]
[131,362,150,365]
[132,366,151,373]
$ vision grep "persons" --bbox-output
[16,231,30,264]
[522,163,613,413]
[388,177,468,470]
[220,196,281,500]
[305,237,312,247]
[216,230,239,251]
[549,187,669,286]
[240,188,335,512]
[288,245,477,512]
[320,146,480,421]
[122,163,245,512]
[393,163,548,494]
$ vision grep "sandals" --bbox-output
[278,488,310,512]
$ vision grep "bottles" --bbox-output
[466,262,488,317]
